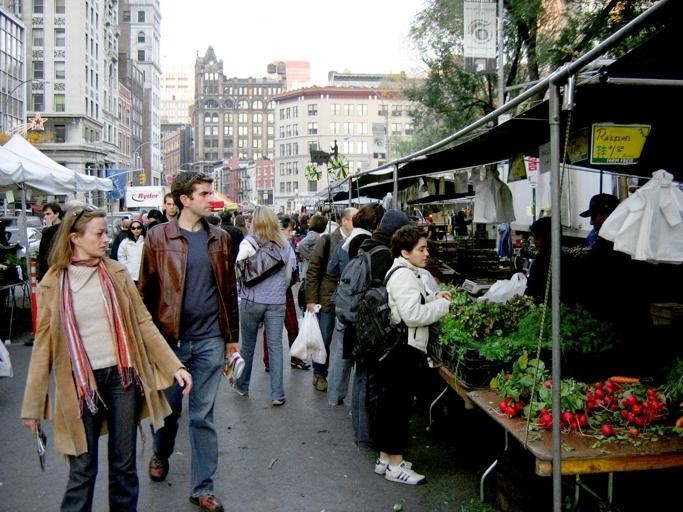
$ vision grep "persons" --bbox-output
[424,216,437,241]
[137,170,239,511]
[299,214,328,279]
[145,210,162,226]
[524,216,575,309]
[19,202,193,512]
[327,203,387,408]
[117,220,144,287]
[232,205,297,405]
[573,194,649,381]
[262,212,311,373]
[373,225,452,485]
[304,208,359,391]
[351,209,409,455]
[36,202,64,283]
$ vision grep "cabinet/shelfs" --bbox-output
[0,280,26,344]
[468,381,683,512]
[422,347,491,425]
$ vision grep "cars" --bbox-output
[106,211,142,248]
[6,227,42,258]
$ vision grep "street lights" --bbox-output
[1,78,51,134]
[131,141,159,187]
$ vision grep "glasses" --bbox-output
[130,226,141,230]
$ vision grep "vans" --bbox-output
[0,216,43,233]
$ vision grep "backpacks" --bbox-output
[333,245,393,327]
[218,211,245,264]
[148,193,178,230]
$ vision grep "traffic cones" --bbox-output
[24,261,37,346]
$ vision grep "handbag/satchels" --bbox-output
[236,235,284,289]
[298,234,331,310]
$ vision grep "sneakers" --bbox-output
[384,460,426,485]
[271,399,285,405]
[290,360,310,370]
[374,457,419,475]
[24,338,34,346]
[312,374,328,391]
[189,494,223,512]
[148,454,169,482]
[230,382,248,396]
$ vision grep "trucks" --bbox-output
[124,184,171,224]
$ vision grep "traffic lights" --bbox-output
[139,173,146,185]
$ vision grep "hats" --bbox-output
[578,193,619,218]
[378,208,408,234]
